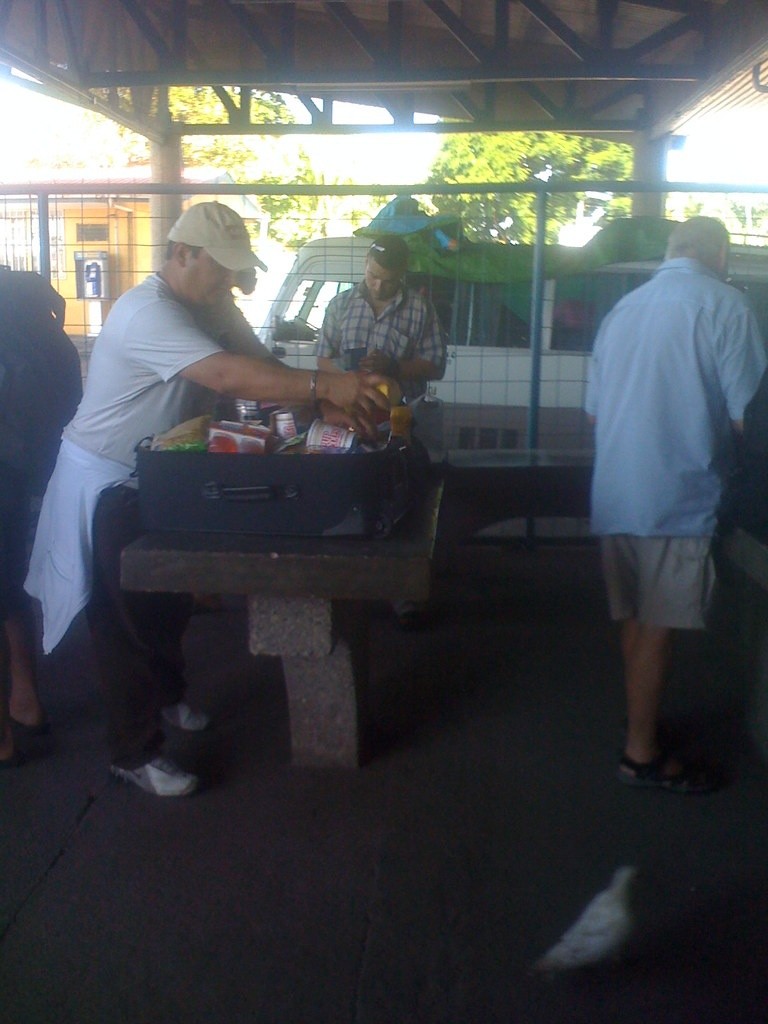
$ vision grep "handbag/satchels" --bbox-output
[401,395,446,464]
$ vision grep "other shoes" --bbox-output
[0,715,56,769]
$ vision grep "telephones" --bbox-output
[85,265,99,280]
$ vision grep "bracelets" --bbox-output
[387,357,400,378]
[310,369,319,405]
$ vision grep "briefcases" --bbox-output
[128,409,431,553]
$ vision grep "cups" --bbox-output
[306,418,359,450]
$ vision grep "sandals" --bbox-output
[616,747,710,792]
[624,718,696,753]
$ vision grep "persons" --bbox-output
[583,216,768,793]
[25,201,448,799]
[0,264,84,767]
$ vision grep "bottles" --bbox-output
[383,405,414,446]
[234,397,258,424]
[275,410,297,438]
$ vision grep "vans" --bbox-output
[233,234,768,456]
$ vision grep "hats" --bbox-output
[371,237,409,270]
[167,202,258,270]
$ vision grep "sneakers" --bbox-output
[111,755,212,797]
[159,691,209,730]
